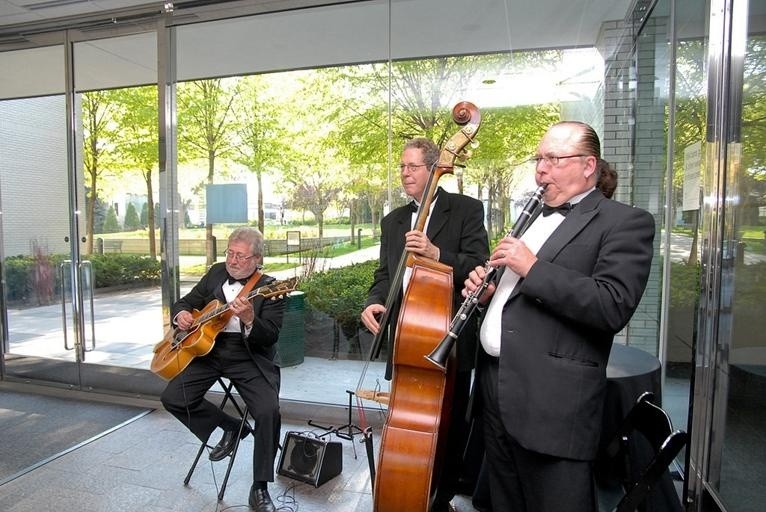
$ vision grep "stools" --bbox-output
[184,374,282,500]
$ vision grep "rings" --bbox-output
[500,250,506,258]
[233,304,238,308]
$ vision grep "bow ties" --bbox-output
[410,203,418,212]
[544,203,570,217]
[229,277,247,285]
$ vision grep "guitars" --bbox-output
[150,277,298,382]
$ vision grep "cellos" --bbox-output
[356,102,481,512]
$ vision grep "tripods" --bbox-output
[315,394,365,459]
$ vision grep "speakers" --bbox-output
[276,431,342,487]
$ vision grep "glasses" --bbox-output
[536,155,582,165]
[224,249,254,260]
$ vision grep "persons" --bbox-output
[160,226,282,512]
[459,117,656,512]
[359,139,490,511]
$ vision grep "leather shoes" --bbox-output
[209,420,250,461]
[250,486,275,512]
